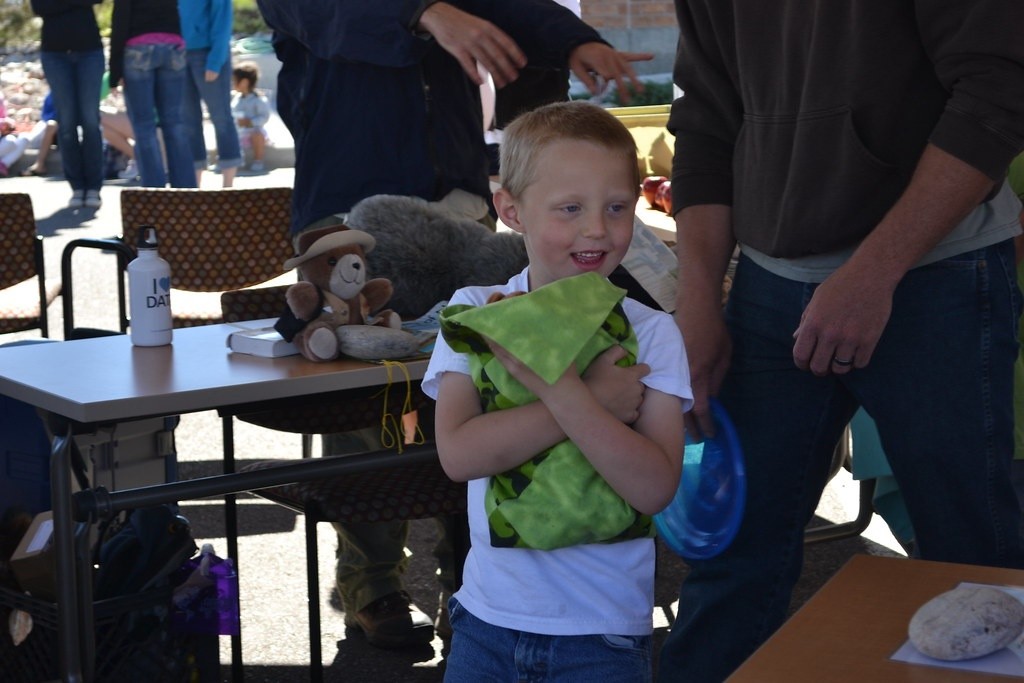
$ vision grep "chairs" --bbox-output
[0,193,49,344]
[223,285,516,683]
[63,186,325,339]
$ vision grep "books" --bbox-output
[226,326,300,359]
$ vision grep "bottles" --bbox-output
[128,226,173,346]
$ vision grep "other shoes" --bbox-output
[67,189,84,209]
[84,189,101,208]
[250,160,265,171]
[118,159,139,179]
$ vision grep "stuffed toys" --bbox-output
[273,223,403,363]
[343,194,529,322]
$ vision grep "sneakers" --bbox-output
[431,605,455,635]
[345,588,434,647]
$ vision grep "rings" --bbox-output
[834,357,853,365]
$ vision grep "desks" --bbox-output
[0,313,459,683]
[721,551,1024,683]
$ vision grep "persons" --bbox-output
[99,78,171,179]
[30,0,105,207]
[21,91,60,176]
[108,0,199,188]
[177,0,242,188]
[421,101,693,683]
[206,66,269,171]
[0,90,31,177]
[657,0,1024,683]
[256,0,654,648]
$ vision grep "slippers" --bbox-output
[18,167,48,176]
